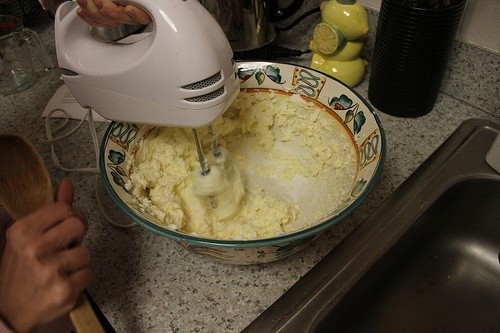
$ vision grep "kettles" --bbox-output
[202,0,306,58]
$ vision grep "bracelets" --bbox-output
[0,313,19,333]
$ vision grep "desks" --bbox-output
[0,9,500,333]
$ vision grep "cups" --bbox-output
[0,27,54,96]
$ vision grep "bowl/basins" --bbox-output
[98,60,385,265]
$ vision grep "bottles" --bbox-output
[368,0,466,119]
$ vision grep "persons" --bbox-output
[0,0,151,333]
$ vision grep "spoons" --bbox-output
[0,134,117,333]
